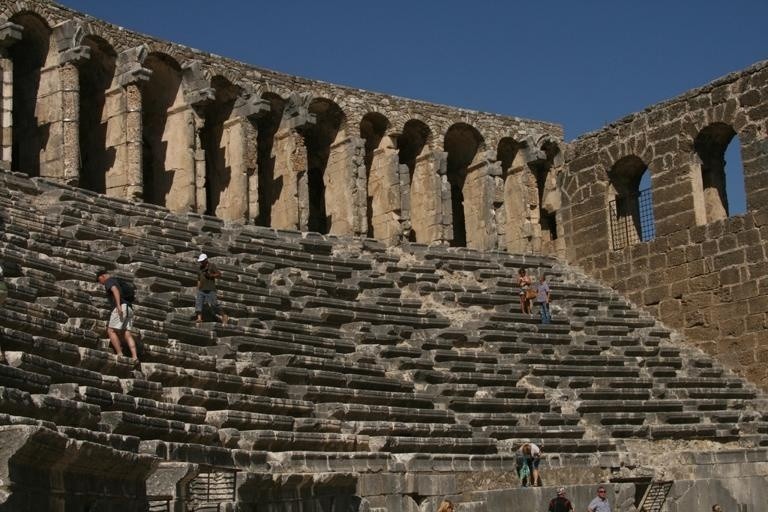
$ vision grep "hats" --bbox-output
[96,270,109,282]
[197,254,207,261]
[557,487,567,494]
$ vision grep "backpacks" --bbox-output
[113,277,137,302]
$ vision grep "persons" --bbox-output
[712,504,723,512]
[587,487,611,512]
[194,254,228,325]
[96,270,139,363]
[438,500,453,512]
[548,488,573,512]
[531,275,551,324]
[518,268,531,314]
[512,443,542,487]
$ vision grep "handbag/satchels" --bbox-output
[525,289,536,299]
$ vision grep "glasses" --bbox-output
[599,491,605,493]
